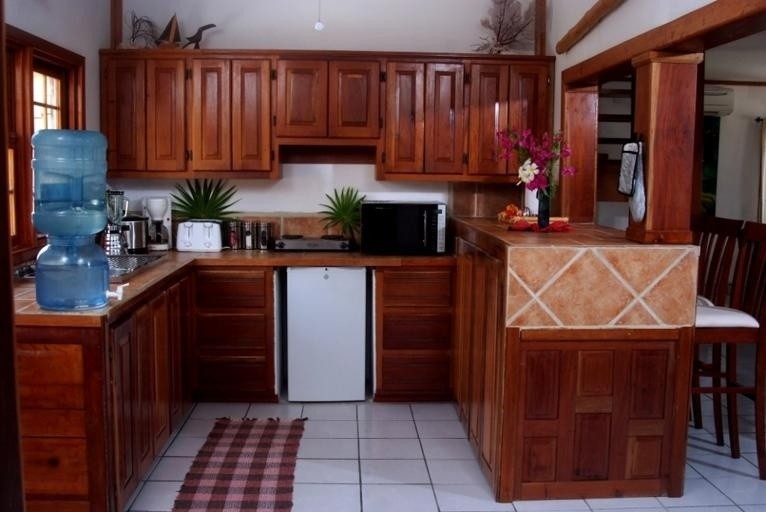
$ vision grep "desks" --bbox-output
[451,214,701,505]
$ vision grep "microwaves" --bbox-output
[360,199,447,255]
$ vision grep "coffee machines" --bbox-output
[141,195,173,251]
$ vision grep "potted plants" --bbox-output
[169,179,242,250]
[317,187,367,252]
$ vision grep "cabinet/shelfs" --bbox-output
[275,50,382,145]
[102,47,187,179]
[195,261,271,402]
[465,53,551,184]
[189,49,282,177]
[385,51,465,180]
[10,261,194,512]
[376,262,453,401]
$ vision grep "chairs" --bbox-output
[687,213,766,480]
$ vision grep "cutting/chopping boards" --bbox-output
[102,255,168,283]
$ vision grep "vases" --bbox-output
[537,184,550,228]
[519,183,539,215]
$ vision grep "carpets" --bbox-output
[171,414,310,511]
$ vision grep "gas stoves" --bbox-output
[274,233,350,251]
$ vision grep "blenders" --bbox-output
[100,190,129,258]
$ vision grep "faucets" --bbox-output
[106,282,130,301]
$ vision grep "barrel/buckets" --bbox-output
[29,128,108,311]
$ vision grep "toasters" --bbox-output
[174,221,222,253]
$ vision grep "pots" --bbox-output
[120,215,149,253]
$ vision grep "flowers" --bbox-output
[469,0,535,54]
[496,128,576,198]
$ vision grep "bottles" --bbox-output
[228,220,272,250]
